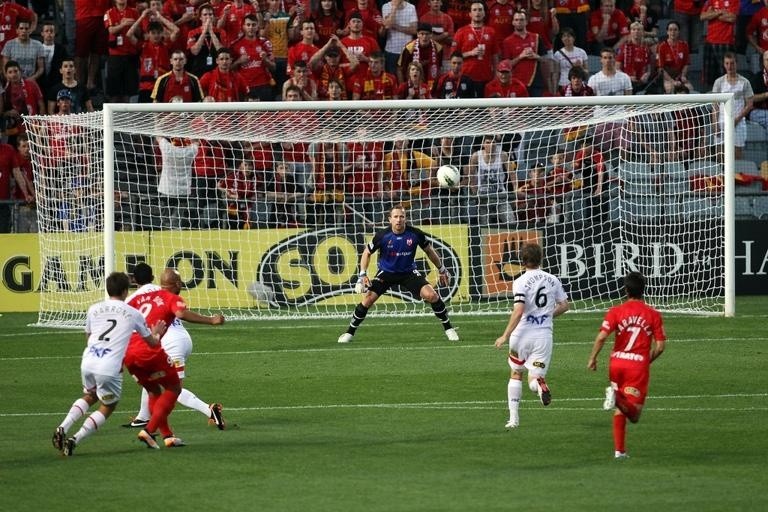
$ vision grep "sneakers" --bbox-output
[337,333,352,343]
[445,328,459,341]
[504,421,519,428]
[536,376,550,406]
[122,420,186,449]
[209,403,225,430]
[602,386,615,411]
[52,428,75,456]
[614,451,629,459]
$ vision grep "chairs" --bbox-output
[515,129,561,180]
[604,160,768,225]
[735,37,761,77]
[742,108,767,160]
[657,18,705,51]
[587,55,602,74]
[688,54,703,90]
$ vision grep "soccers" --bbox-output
[437,165,461,190]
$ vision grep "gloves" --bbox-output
[354,271,371,294]
[438,265,450,287]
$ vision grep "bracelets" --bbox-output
[438,263,445,273]
[359,271,366,277]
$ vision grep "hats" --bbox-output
[326,48,338,57]
[497,60,512,72]
[417,24,432,32]
[57,89,72,101]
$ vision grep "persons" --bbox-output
[125,263,226,429]
[338,205,460,343]
[588,272,668,457]
[53,271,167,457]
[496,243,569,428]
[122,268,225,450]
